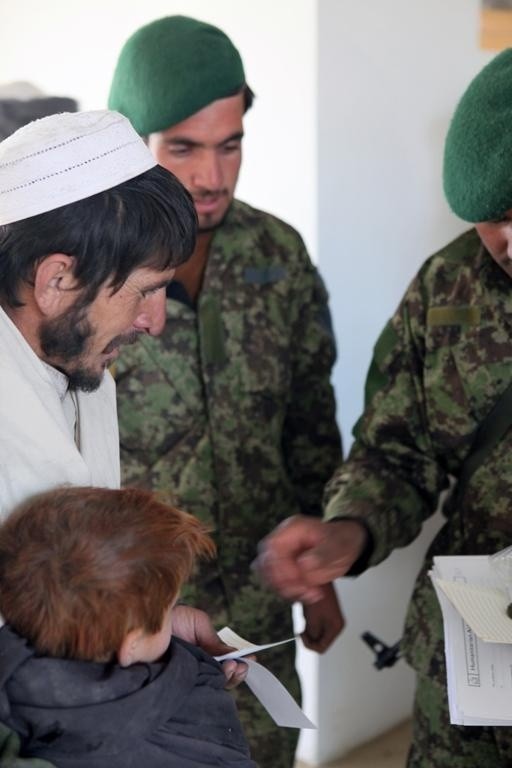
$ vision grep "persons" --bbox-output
[108,14,345,768]
[0,109,258,691]
[247,46,512,768]
[0,485,259,768]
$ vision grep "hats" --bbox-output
[439,45,511,224]
[110,14,254,140]
[0,106,159,227]
